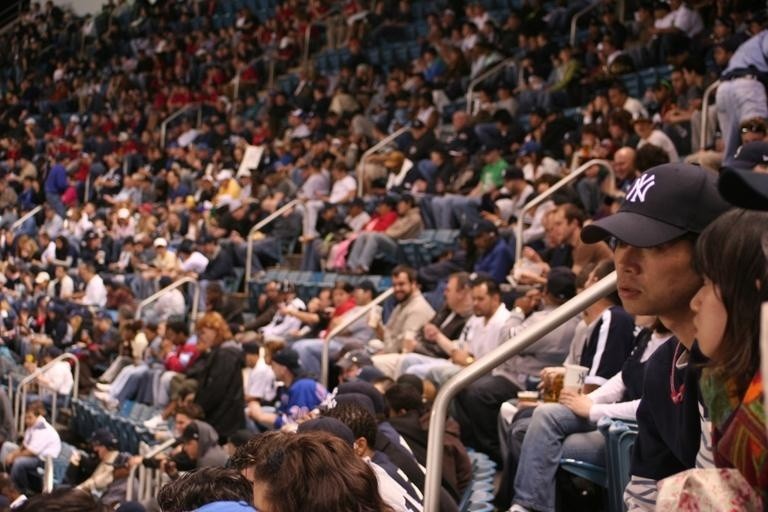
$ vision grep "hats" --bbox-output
[289,107,305,119]
[547,264,578,299]
[34,270,50,283]
[216,168,231,180]
[331,383,386,412]
[152,237,169,248]
[296,416,355,451]
[332,392,376,416]
[342,365,396,383]
[336,352,374,369]
[627,111,653,124]
[729,141,768,167]
[271,350,306,376]
[466,218,497,237]
[578,163,723,249]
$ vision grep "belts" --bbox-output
[720,65,762,81]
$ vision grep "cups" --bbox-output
[543,369,564,404]
[560,360,590,399]
